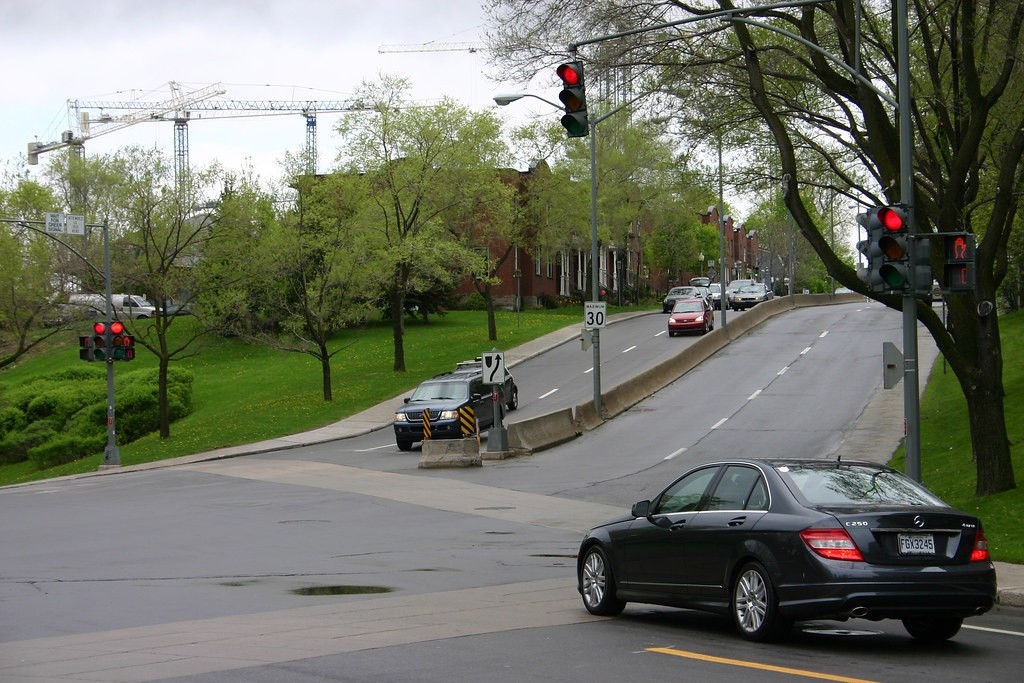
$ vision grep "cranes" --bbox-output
[377,24,694,156]
[26,78,450,281]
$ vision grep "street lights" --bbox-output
[493,85,694,413]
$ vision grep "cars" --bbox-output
[666,298,715,336]
[575,459,999,646]
[662,276,774,313]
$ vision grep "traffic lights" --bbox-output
[941,234,977,290]
[92,321,107,361]
[124,335,135,361]
[555,62,589,138]
[854,206,886,293]
[78,336,94,362]
[108,322,124,360]
[878,204,911,291]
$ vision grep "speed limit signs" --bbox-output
[582,300,607,332]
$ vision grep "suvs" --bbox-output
[453,353,520,410]
[391,372,506,453]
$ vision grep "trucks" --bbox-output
[41,292,168,325]
